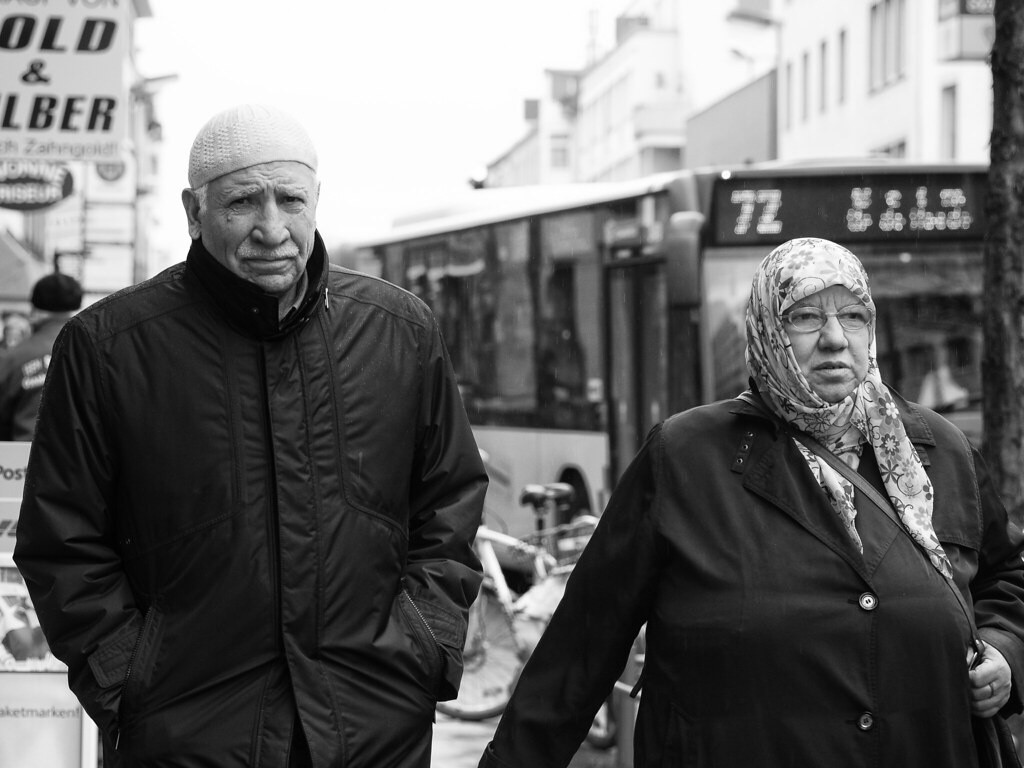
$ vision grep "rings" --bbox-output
[989,683,994,698]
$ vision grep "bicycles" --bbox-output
[438,448,621,749]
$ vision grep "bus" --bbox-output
[323,156,993,590]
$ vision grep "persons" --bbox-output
[0,272,83,443]
[14,108,489,768]
[479,237,1024,768]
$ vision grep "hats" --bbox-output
[187,103,319,188]
[30,274,83,311]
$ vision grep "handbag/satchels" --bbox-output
[974,713,1023,768]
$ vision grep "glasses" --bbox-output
[781,305,872,334]
